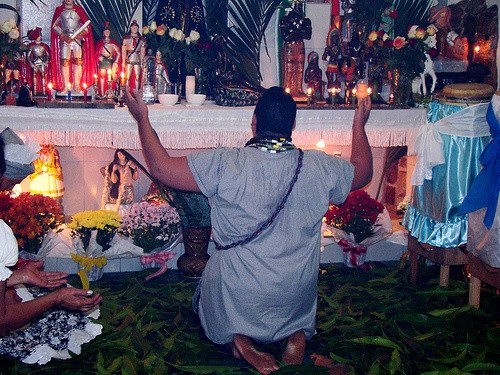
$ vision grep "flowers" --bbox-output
[0,189,66,262]
[0,18,42,63]
[365,24,435,108]
[67,210,122,282]
[396,195,410,226]
[142,21,200,74]
[118,200,183,281]
[323,190,393,268]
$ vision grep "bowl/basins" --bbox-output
[158,94,178,105]
[186,94,206,106]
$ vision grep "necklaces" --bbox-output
[118,160,129,168]
[354,42,361,51]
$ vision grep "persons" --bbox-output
[124,86,373,375]
[0,134,103,365]
[0,0,171,107]
[109,149,156,212]
[305,26,387,105]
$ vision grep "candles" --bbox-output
[48,82,52,102]
[107,69,111,82]
[285,87,290,94]
[332,87,335,107]
[352,80,372,106]
[120,72,125,87]
[93,74,98,87]
[186,76,195,100]
[307,87,311,106]
[68,83,71,104]
[83,83,88,103]
